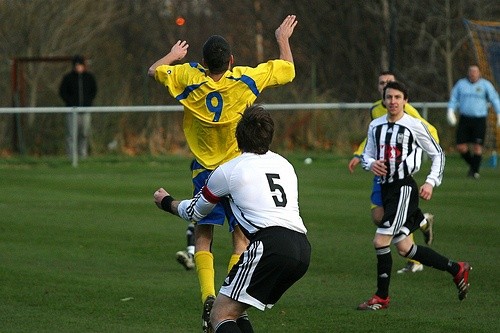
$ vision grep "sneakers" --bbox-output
[357,294,389,311]
[452,262,473,301]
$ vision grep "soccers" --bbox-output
[304,158,312,164]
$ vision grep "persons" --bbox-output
[148,15,297,333]
[58,54,99,167]
[175,157,215,271]
[153,104,312,333]
[348,71,439,275]
[358,80,473,311]
[446,63,500,179]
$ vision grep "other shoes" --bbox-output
[396,260,423,273]
[420,213,436,246]
[202,297,216,333]
[175,250,196,270]
